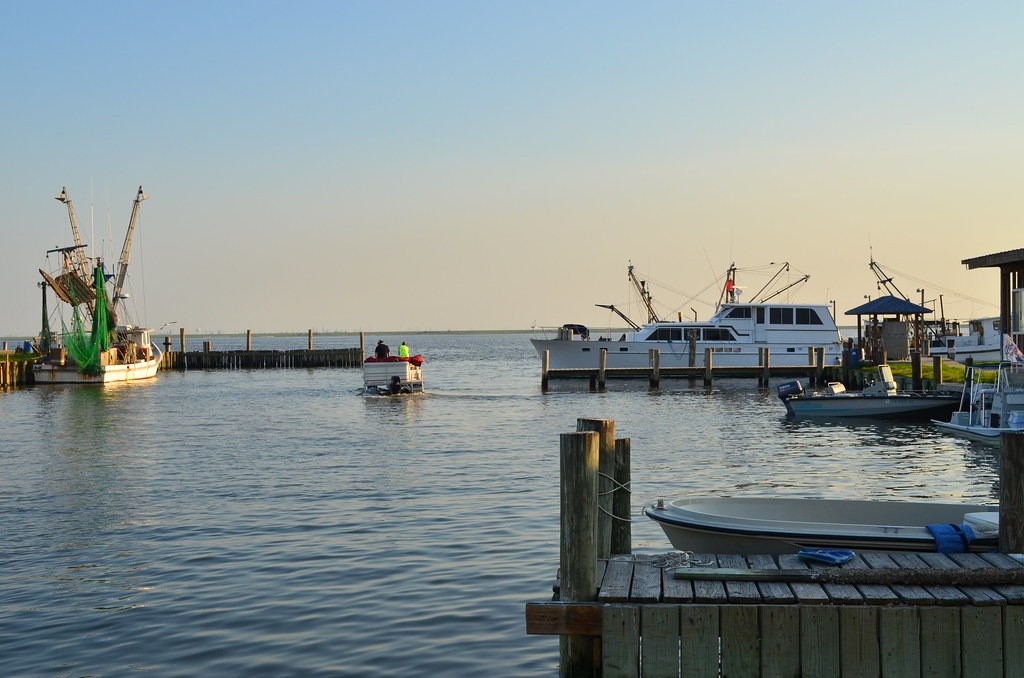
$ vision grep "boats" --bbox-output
[909,316,1001,363]
[776,364,961,417]
[930,363,1024,447]
[843,319,921,347]
[363,357,424,394]
[642,493,999,554]
[528,303,845,378]
[31,323,163,383]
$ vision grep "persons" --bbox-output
[398,342,410,357]
[373,340,390,359]
